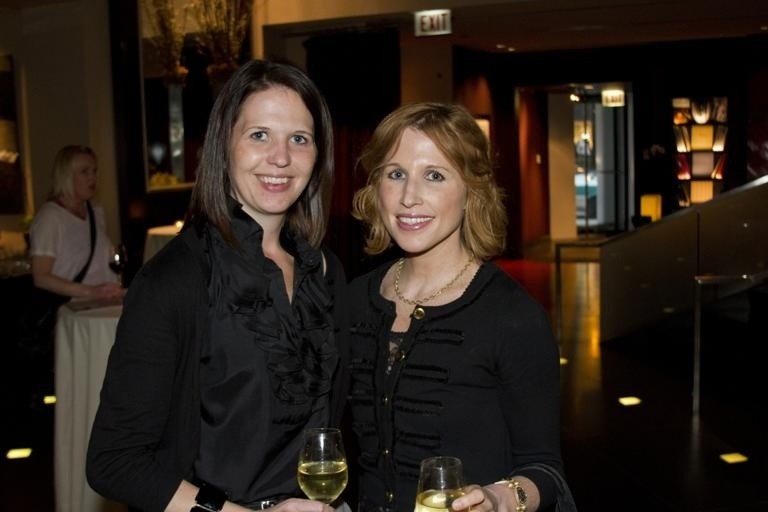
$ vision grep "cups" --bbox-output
[413,456,470,511]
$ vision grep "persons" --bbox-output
[341,99,578,511]
[83,59,360,511]
[28,143,129,306]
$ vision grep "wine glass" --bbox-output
[107,244,128,288]
[294,427,350,505]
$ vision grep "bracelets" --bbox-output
[188,483,228,510]
[63,280,93,301]
[497,475,531,511]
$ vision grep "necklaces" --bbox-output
[392,255,480,323]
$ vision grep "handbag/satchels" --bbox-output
[14,303,57,358]
